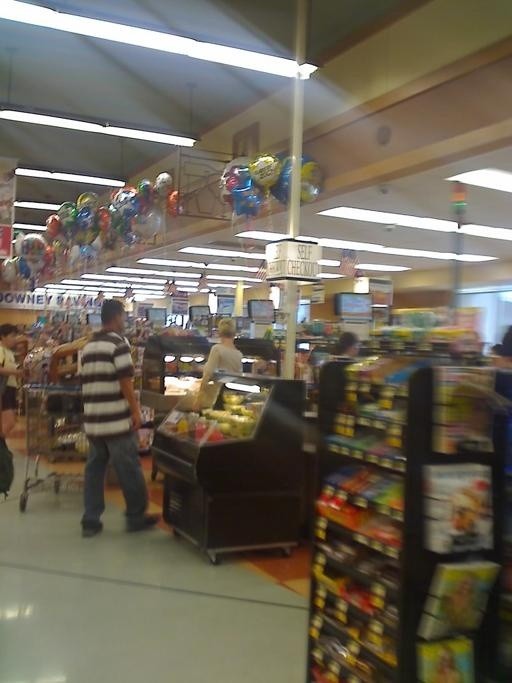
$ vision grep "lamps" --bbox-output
[1,1,318,80]
[177,247,414,272]
[444,168,511,192]
[1,47,201,147]
[12,223,47,231]
[26,267,317,297]
[14,137,125,187]
[12,240,16,243]
[316,206,511,241]
[234,230,500,262]
[135,258,347,278]
[14,201,62,211]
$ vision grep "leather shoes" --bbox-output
[81,521,105,536]
[127,514,161,533]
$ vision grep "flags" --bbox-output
[170,280,177,294]
[96,291,105,300]
[162,280,170,295]
[196,274,208,292]
[124,287,133,299]
[337,249,360,279]
[64,293,89,307]
[256,259,267,282]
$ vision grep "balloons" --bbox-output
[216,146,325,216]
[1,170,186,291]
[22,345,53,376]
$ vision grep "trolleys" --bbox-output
[20,372,90,511]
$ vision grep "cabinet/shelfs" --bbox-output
[150,371,305,564]
[304,358,512,683]
[137,334,308,482]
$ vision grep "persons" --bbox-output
[335,329,359,358]
[80,300,161,538]
[488,325,511,366]
[1,320,27,439]
[194,315,244,410]
